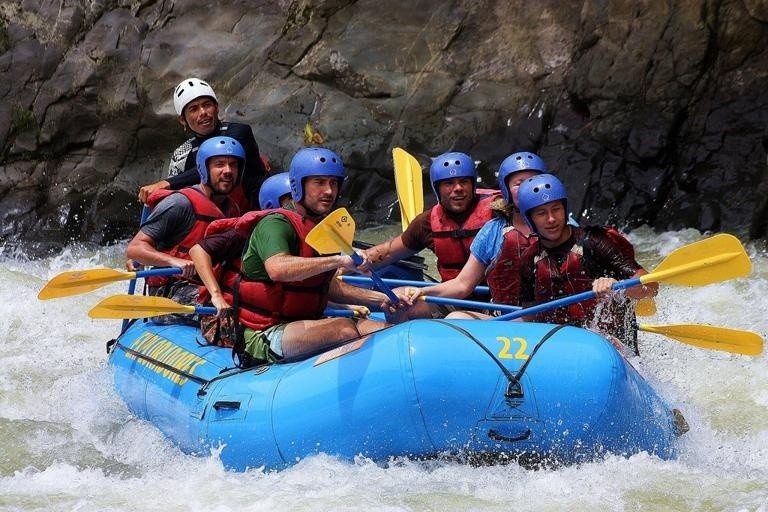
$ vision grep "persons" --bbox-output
[126,78,272,272]
[403,151,579,321]
[334,152,505,325]
[232,147,413,370]
[189,171,371,349]
[126,136,246,328]
[509,173,659,357]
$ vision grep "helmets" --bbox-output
[429,152,477,205]
[497,152,547,207]
[258,172,292,211]
[173,78,218,117]
[195,136,247,186]
[516,173,570,235]
[288,147,347,204]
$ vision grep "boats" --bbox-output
[107,312,682,477]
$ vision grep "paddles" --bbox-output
[87,295,360,320]
[305,208,401,308]
[37,267,183,299]
[483,231,752,321]
[404,288,763,356]
